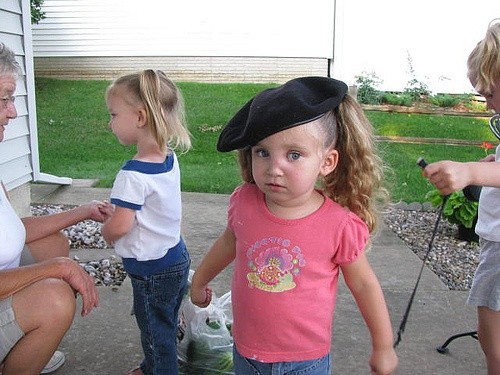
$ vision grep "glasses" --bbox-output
[0,96,15,106]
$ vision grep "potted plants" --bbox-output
[424,187,480,242]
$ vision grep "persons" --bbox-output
[0,44,116,375]
[190,76,399,375]
[97,69,191,375]
[422,18,500,375]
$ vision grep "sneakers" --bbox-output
[40,351,65,373]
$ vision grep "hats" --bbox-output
[217,76,348,152]
